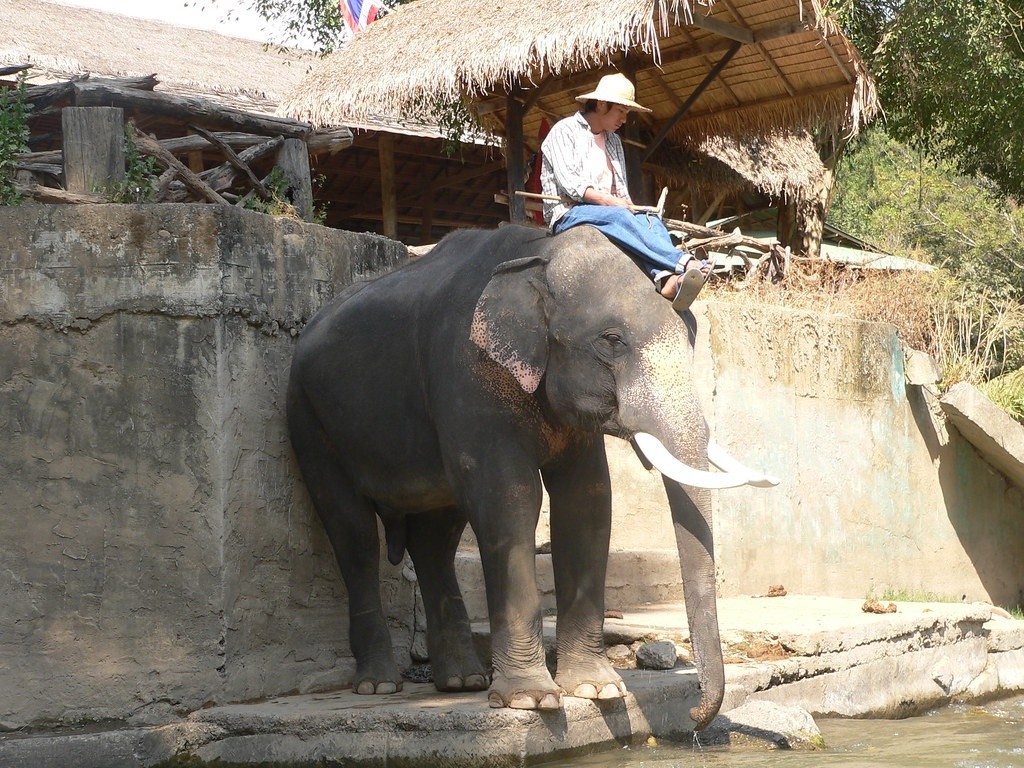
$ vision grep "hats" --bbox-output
[574,72,653,113]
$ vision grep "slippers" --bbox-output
[672,267,705,312]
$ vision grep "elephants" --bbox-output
[282,220,783,734]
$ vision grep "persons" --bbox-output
[542,72,717,312]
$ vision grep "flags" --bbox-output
[339,0,377,37]
